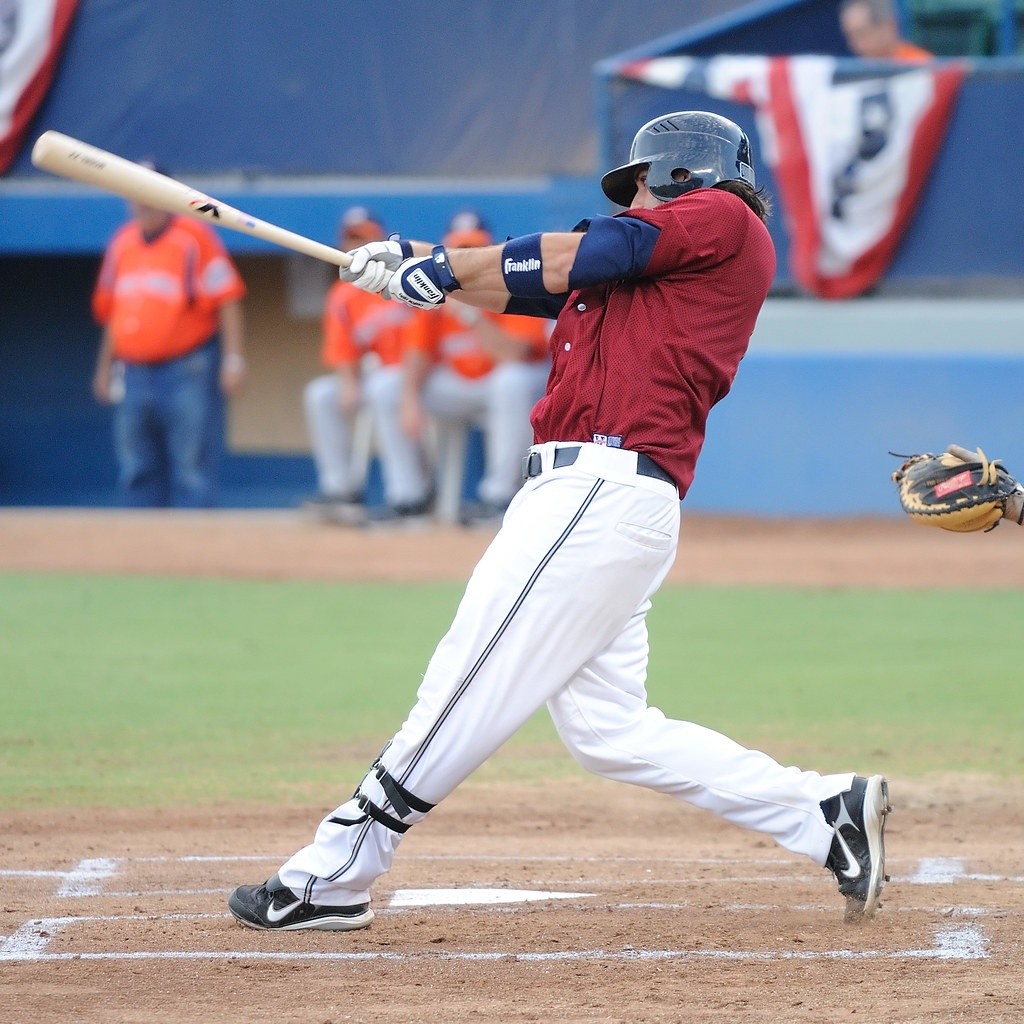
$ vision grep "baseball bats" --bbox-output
[29,128,396,287]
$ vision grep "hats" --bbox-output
[139,157,173,178]
[444,212,490,235]
[342,207,386,233]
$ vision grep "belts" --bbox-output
[523,446,676,488]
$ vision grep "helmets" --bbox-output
[600,111,755,208]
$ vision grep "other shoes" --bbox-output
[301,492,365,523]
[459,499,511,525]
[371,489,436,522]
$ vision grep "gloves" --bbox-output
[388,244,462,310]
[339,233,414,295]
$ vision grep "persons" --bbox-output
[92,160,248,507]
[839,0,934,60]
[301,207,550,520]
[891,437,1024,547]
[228,110,892,931]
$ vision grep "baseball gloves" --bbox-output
[892,444,1018,533]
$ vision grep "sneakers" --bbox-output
[820,776,893,926]
[228,873,374,931]
